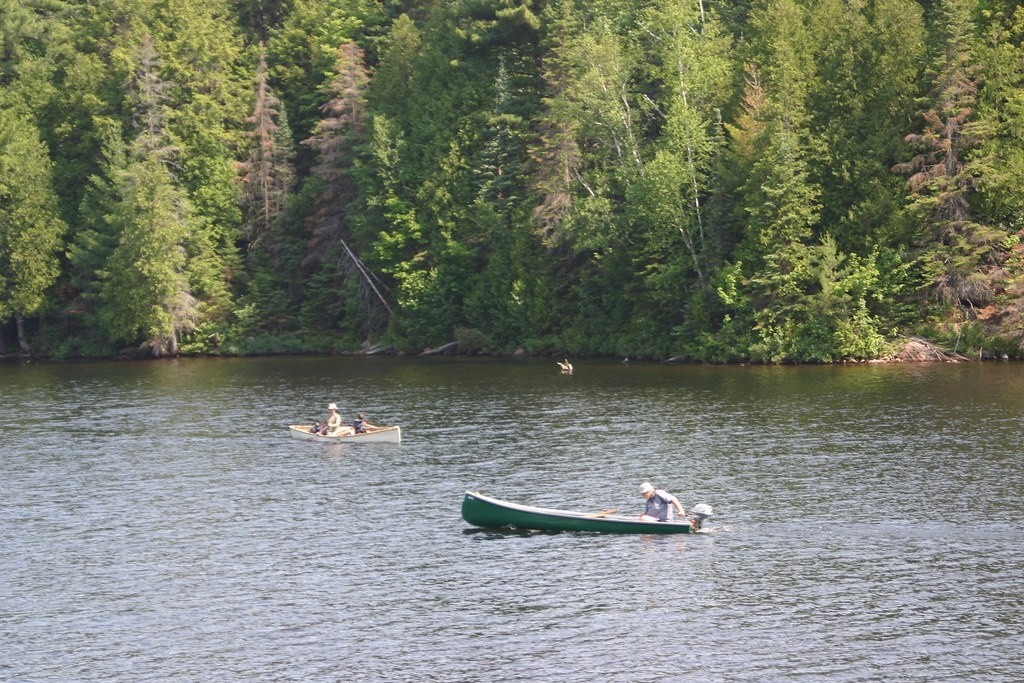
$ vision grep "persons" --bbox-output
[638,483,684,521]
[317,403,340,435]
[354,413,378,434]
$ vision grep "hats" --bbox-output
[328,403,338,410]
[638,482,654,494]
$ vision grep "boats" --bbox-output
[460,490,714,533]
[557,361,573,374]
[288,424,401,445]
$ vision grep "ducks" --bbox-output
[556,359,573,371]
